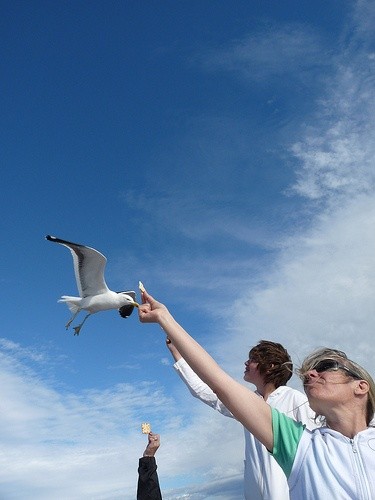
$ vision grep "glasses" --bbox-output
[312,359,362,380]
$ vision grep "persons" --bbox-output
[166,337,327,500]
[137,289,375,500]
[136,432,162,500]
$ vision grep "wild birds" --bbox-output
[45,235,139,336]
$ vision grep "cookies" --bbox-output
[139,281,145,292]
[142,423,150,434]
